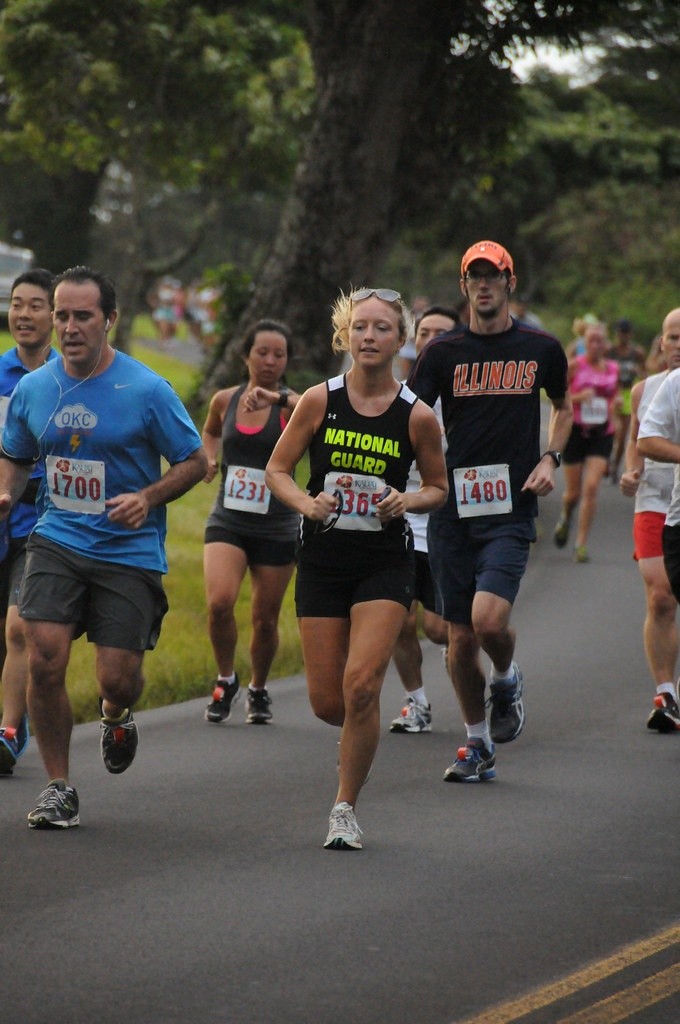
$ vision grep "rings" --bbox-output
[392,512,396,518]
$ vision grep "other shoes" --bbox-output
[553,519,570,549]
[575,542,589,562]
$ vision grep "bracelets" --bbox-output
[278,390,287,407]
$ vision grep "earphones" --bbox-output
[105,319,110,331]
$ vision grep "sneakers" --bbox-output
[443,736,496,782]
[99,696,138,774]
[647,692,680,733]
[245,687,272,719]
[389,697,432,733]
[485,659,525,743]
[205,672,241,722]
[18,714,29,758]
[324,802,363,850]
[0,728,18,774]
[606,466,621,485]
[28,778,80,828]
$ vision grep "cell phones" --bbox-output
[379,486,391,502]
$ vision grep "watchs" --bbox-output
[546,451,561,467]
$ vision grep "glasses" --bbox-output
[313,489,343,536]
[464,271,508,282]
[350,289,402,312]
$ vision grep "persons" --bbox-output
[548,315,646,562]
[150,276,222,347]
[200,319,303,723]
[263,287,448,849]
[405,241,574,781]
[620,308,680,734]
[0,270,61,776]
[341,295,545,732]
[0,266,207,829]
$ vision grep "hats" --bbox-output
[461,240,513,276]
